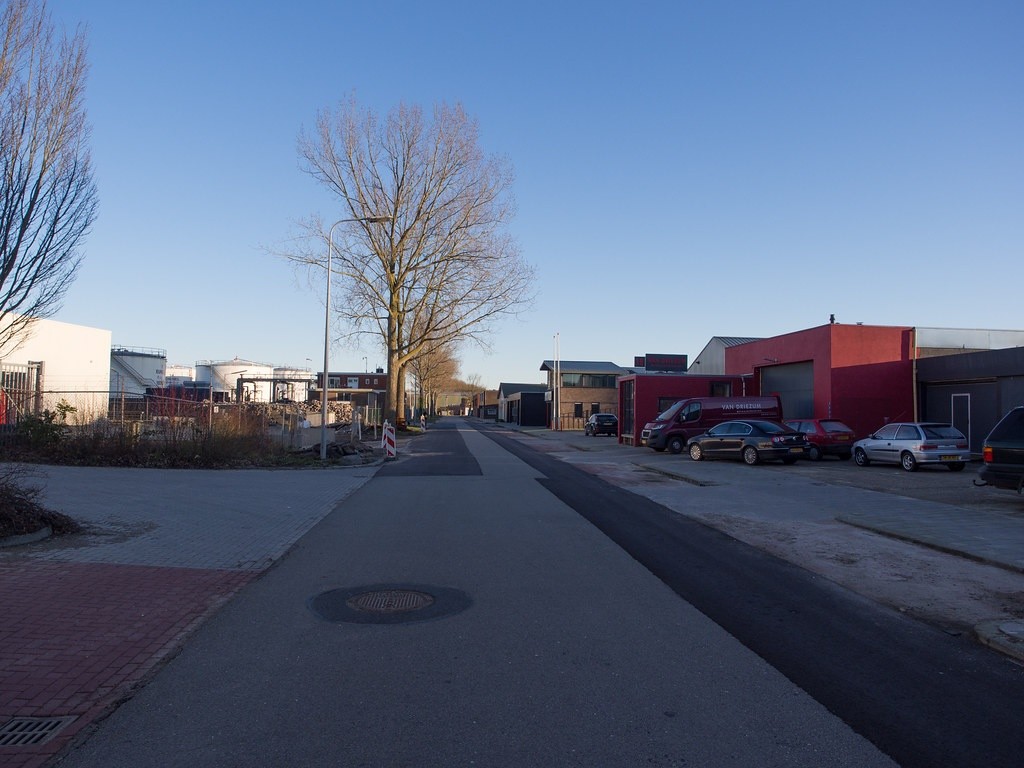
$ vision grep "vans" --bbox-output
[639,392,785,454]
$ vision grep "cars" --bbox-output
[851,423,971,472]
[979,406,1024,496]
[584,413,618,437]
[686,417,812,467]
[775,417,857,463]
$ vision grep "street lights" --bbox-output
[319,215,392,462]
[414,350,437,428]
